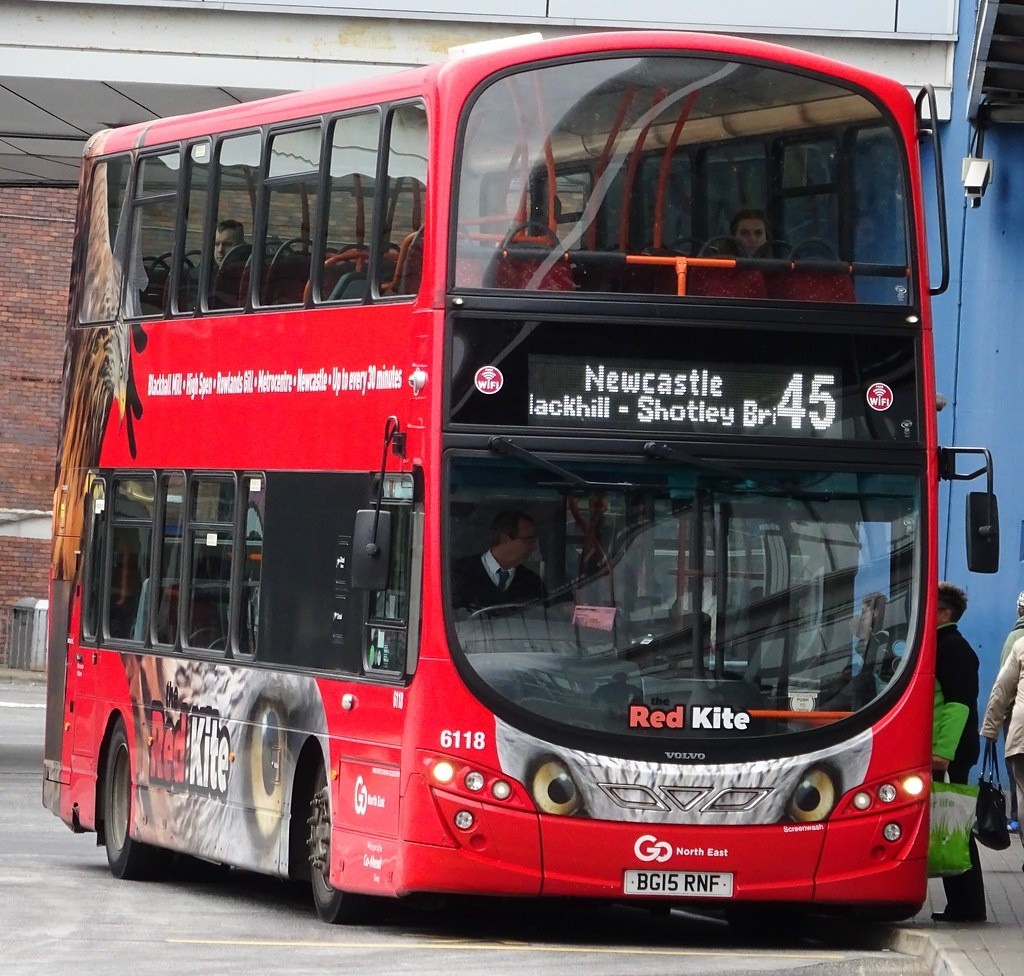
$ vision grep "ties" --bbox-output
[497,570,510,591]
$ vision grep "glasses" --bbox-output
[516,536,536,547]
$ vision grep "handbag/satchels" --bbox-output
[926,768,980,877]
[969,738,1011,850]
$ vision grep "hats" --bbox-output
[1017,590,1024,609]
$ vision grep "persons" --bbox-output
[856,593,889,671]
[927,581,988,922]
[449,504,550,622]
[979,591,1024,872]
[180,220,246,311]
[731,209,768,261]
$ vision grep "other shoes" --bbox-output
[1005,820,1020,833]
[931,913,986,923]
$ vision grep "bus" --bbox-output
[157,536,263,630]
[41,30,1000,945]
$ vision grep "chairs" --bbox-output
[96,549,252,655]
[125,218,861,305]
